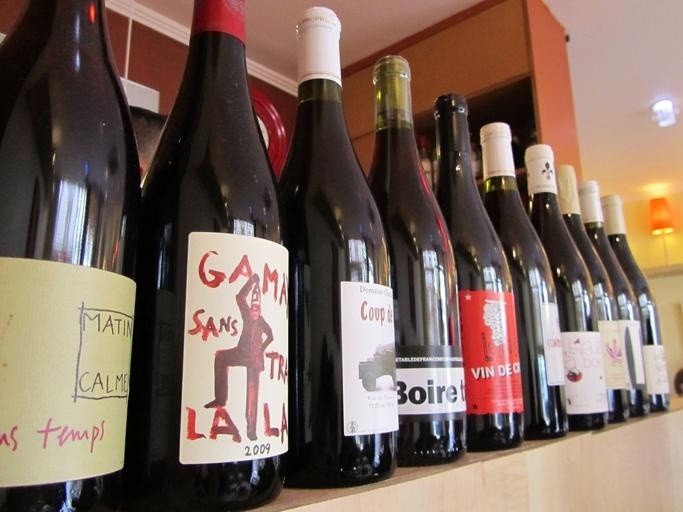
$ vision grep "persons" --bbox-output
[204,274,275,441]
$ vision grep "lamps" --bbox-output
[649,198,675,265]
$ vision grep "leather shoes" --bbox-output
[248,433,256,440]
[205,401,224,407]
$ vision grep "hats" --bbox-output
[251,284,261,304]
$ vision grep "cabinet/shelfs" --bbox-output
[341,1,582,216]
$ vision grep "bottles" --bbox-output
[479,120,572,441]
[559,164,633,422]
[429,91,526,451]
[367,54,470,468]
[524,143,611,434]
[274,4,400,491]
[121,1,292,512]
[2,0,142,512]
[603,193,672,413]
[579,179,651,421]
